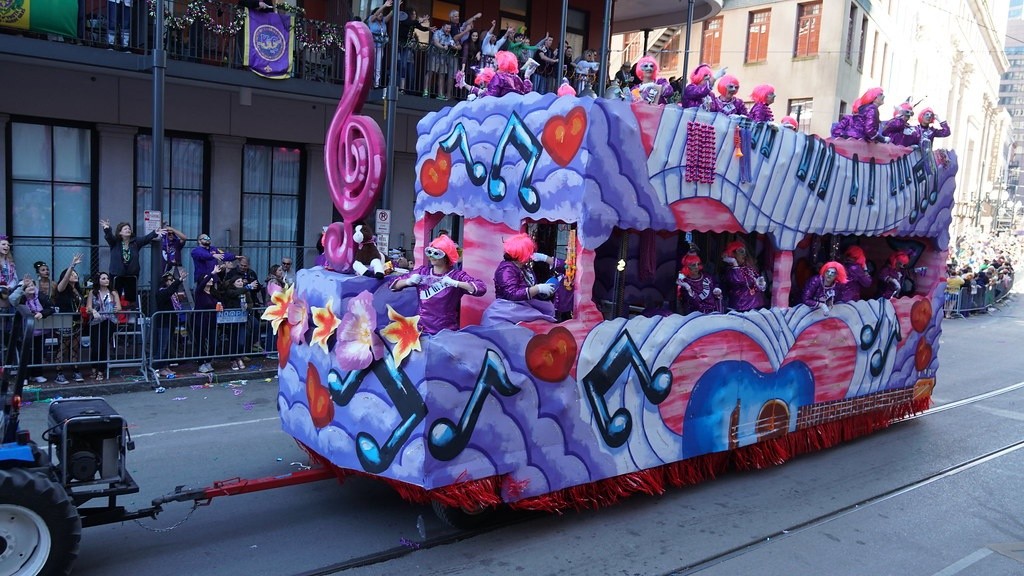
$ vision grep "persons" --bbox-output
[746,85,777,122]
[103,0,132,54]
[781,116,799,131]
[456,49,540,101]
[804,261,846,316]
[191,232,296,372]
[722,242,767,310]
[389,235,487,334]
[616,62,687,93]
[624,55,674,104]
[0,235,123,386]
[916,108,951,148]
[675,252,723,314]
[682,65,727,111]
[158,226,190,376]
[480,232,567,327]
[715,72,749,115]
[944,235,1024,318]
[238,0,274,13]
[371,0,600,101]
[836,246,871,301]
[100,217,169,323]
[833,88,914,144]
[877,250,928,299]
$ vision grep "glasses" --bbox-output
[166,277,175,282]
[200,236,210,241]
[282,261,290,265]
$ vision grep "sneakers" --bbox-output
[71,372,85,381]
[23,379,28,385]
[237,360,246,369]
[231,361,239,370]
[53,374,70,385]
[198,364,210,373]
[30,376,47,382]
[205,363,215,372]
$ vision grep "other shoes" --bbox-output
[423,91,429,98]
[266,353,278,360]
[253,345,264,351]
[159,369,177,375]
[241,356,251,361]
[437,94,448,100]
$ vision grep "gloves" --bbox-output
[537,283,555,297]
[441,276,459,288]
[406,274,421,287]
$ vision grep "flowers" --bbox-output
[148,0,345,53]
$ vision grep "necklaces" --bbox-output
[740,264,767,295]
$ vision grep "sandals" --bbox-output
[96,373,104,381]
[90,372,96,379]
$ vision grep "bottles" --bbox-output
[112,302,116,313]
[217,302,221,311]
